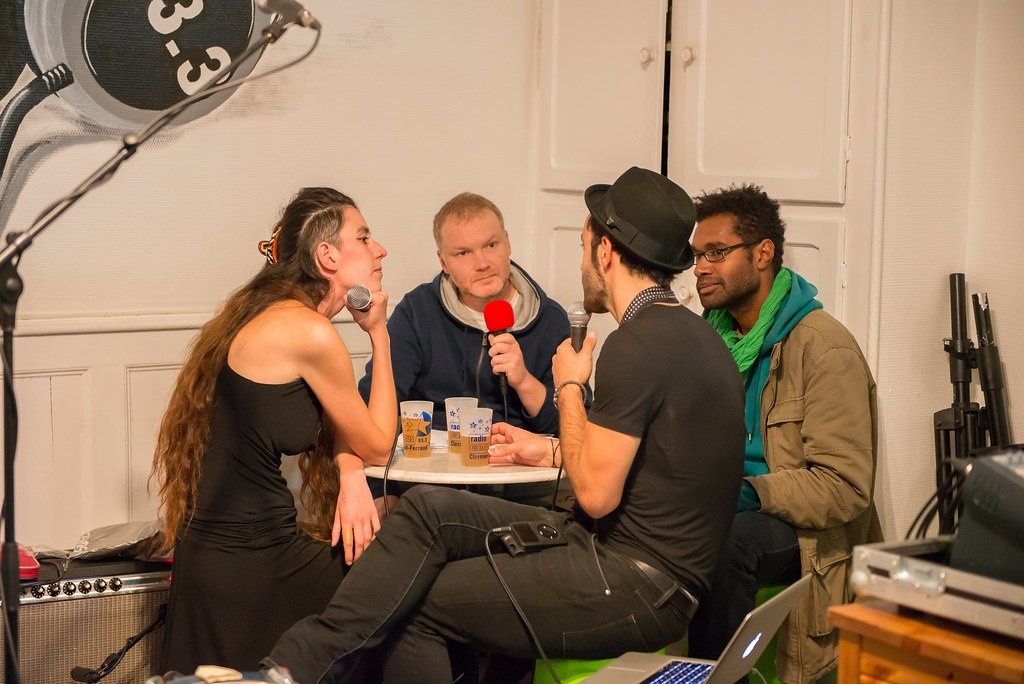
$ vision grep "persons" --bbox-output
[359,192,571,440]
[145,187,398,677]
[688,183,887,683]
[198,164,748,684]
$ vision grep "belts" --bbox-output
[622,553,699,621]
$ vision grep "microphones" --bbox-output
[255,0,321,31]
[484,300,514,396]
[567,301,592,352]
[347,285,372,312]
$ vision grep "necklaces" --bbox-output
[620,285,680,326]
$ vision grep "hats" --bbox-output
[584,166,694,271]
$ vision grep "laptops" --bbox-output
[578,571,814,684]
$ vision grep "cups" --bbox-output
[459,407,493,466]
[399,400,435,458]
[444,397,479,455]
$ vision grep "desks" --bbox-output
[364,446,568,484]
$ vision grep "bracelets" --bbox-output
[553,380,587,410]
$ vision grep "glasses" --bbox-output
[694,240,760,265]
